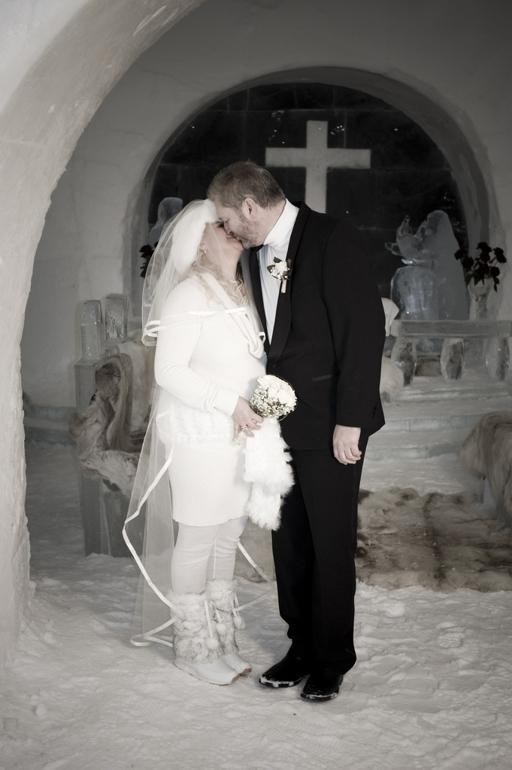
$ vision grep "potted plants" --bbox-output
[454,240,508,320]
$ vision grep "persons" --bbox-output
[149,196,184,245]
[148,196,277,685]
[204,160,385,703]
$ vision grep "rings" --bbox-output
[241,426,247,429]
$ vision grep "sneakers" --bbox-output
[258,640,309,688]
[303,666,343,702]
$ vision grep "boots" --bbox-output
[168,585,253,686]
